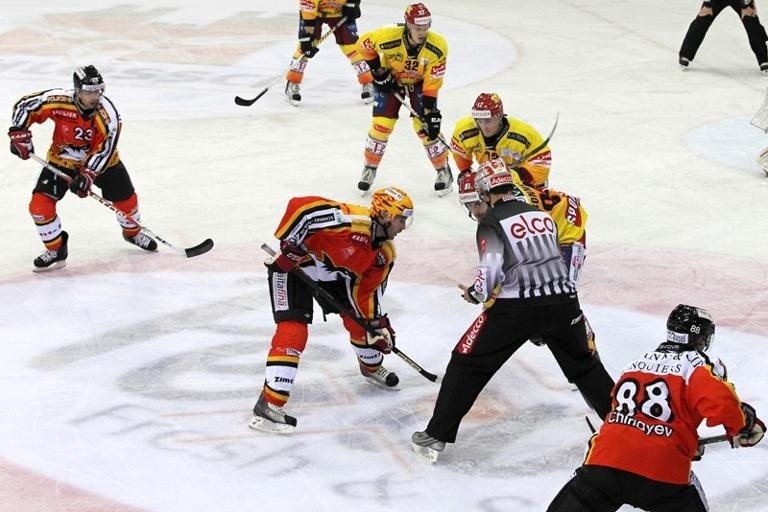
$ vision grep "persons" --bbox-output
[408,155,618,452]
[545,302,768,512]
[453,90,554,184]
[678,0,768,68]
[8,65,159,269]
[457,171,602,391]
[354,2,455,192]
[285,0,382,102]
[249,174,414,427]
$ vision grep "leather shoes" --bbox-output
[298,33,313,56]
[421,110,442,139]
[723,403,766,448]
[360,313,395,355]
[692,436,703,461]
[263,242,309,275]
[8,127,34,159]
[69,166,97,198]
[342,0,361,23]
[372,69,399,94]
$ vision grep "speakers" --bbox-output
[123,231,157,251]
[34,232,68,267]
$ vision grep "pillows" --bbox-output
[371,187,413,221]
[403,3,433,26]
[667,304,715,354]
[472,92,504,119]
[459,159,513,202]
[73,65,104,96]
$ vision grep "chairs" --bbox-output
[362,82,374,98]
[285,80,301,101]
[360,365,399,387]
[253,393,296,427]
[358,166,375,190]
[434,166,452,191]
[412,431,445,452]
